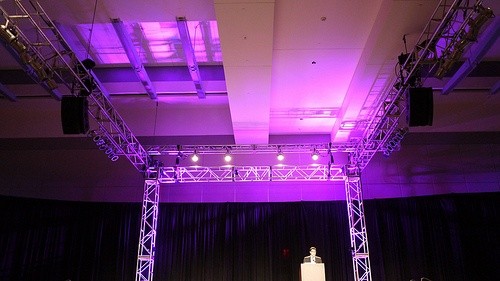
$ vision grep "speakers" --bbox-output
[404,86,434,127]
[61,95,90,135]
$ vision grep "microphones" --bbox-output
[313,260,314,261]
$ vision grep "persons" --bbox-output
[303,247,322,263]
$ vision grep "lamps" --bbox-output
[311,152,318,160]
[277,152,284,160]
[224,153,231,162]
[191,154,199,162]
